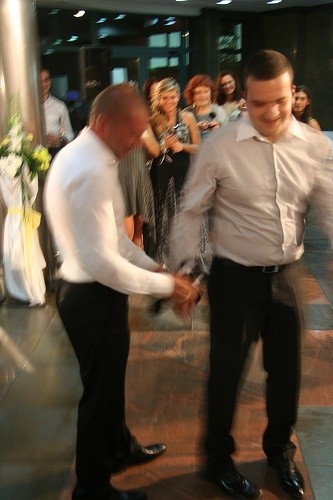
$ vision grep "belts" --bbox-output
[216,257,296,274]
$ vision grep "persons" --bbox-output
[39,67,321,317]
[168,49,333,500]
[42,78,196,500]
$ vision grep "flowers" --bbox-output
[0,88,52,217]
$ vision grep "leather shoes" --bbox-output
[112,443,167,474]
[207,465,263,500]
[72,487,146,500]
[267,457,305,499]
